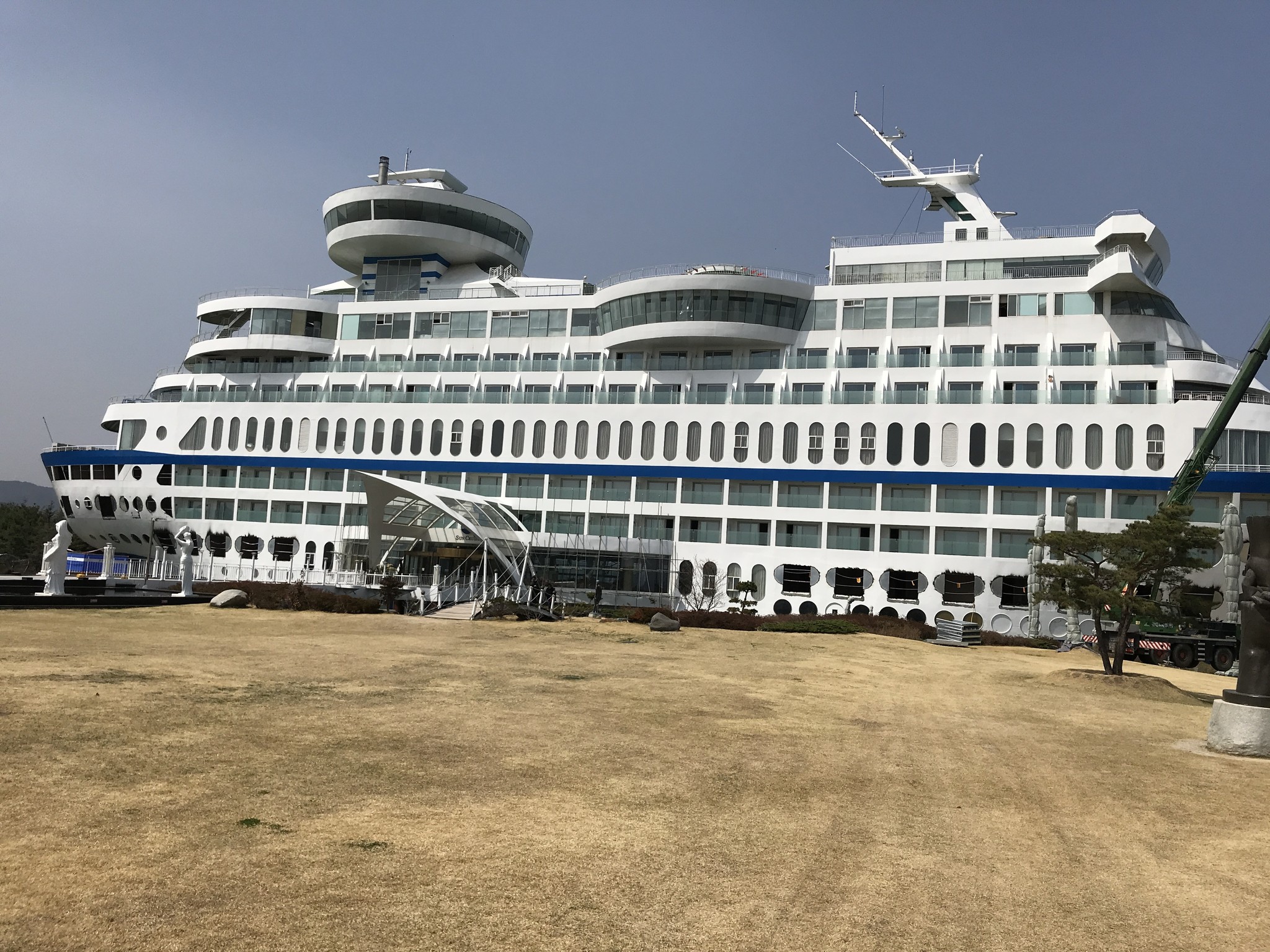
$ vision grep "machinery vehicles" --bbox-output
[1081,313,1270,671]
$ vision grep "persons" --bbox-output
[42,520,73,593]
[593,580,602,612]
[174,528,194,596]
[531,575,557,612]
